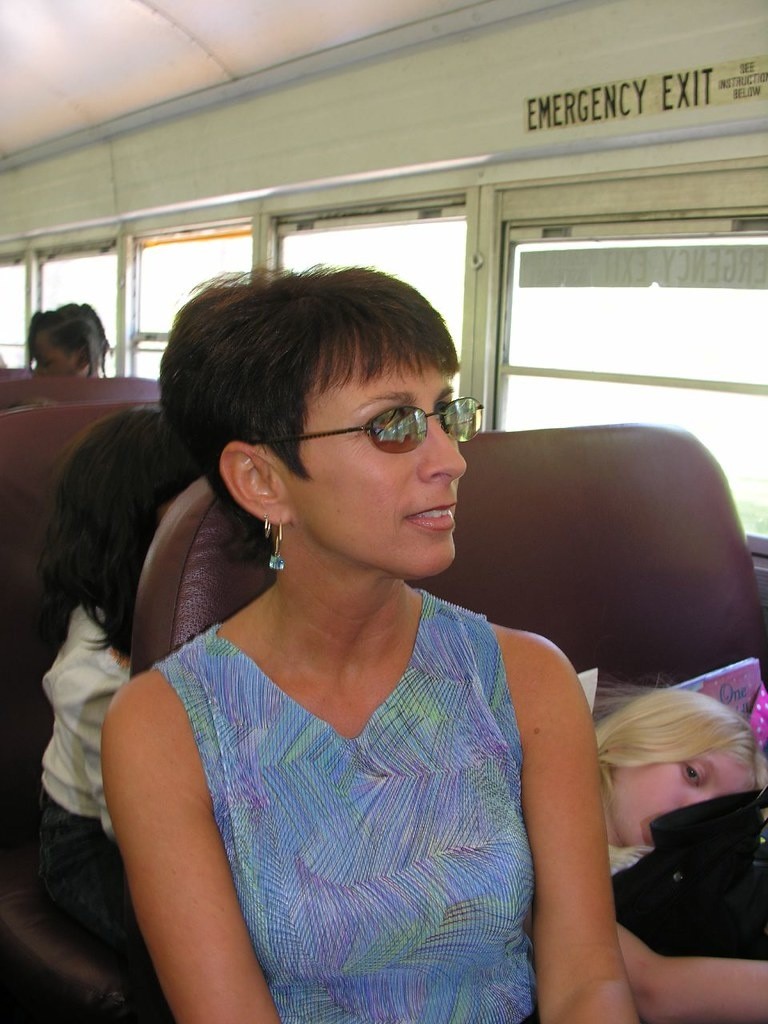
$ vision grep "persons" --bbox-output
[29,302,109,375]
[99,267,638,1024]
[33,407,200,941]
[594,688,768,1023]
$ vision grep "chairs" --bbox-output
[125,420,767,1024]
[1,406,168,1003]
[1,375,159,409]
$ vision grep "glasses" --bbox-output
[248,397,484,455]
